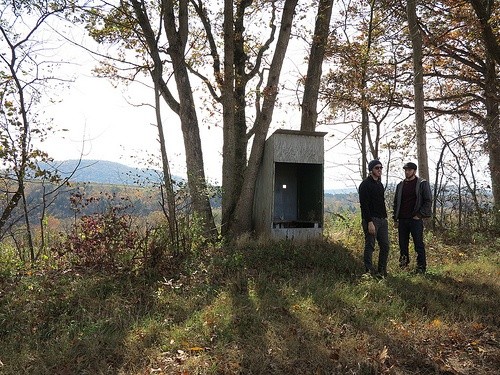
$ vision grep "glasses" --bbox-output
[372,165,383,170]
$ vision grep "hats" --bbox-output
[403,163,417,169]
[367,160,381,171]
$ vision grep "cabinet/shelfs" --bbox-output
[251,127,328,246]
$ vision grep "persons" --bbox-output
[392,163,432,275]
[358,160,389,276]
